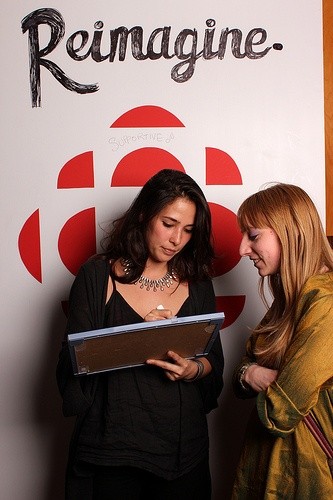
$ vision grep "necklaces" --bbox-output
[121,258,175,292]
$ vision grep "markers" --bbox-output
[157,305,164,310]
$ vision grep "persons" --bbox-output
[232,182,333,500]
[55,170,224,500]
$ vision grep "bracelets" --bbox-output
[183,359,204,383]
[237,362,257,390]
